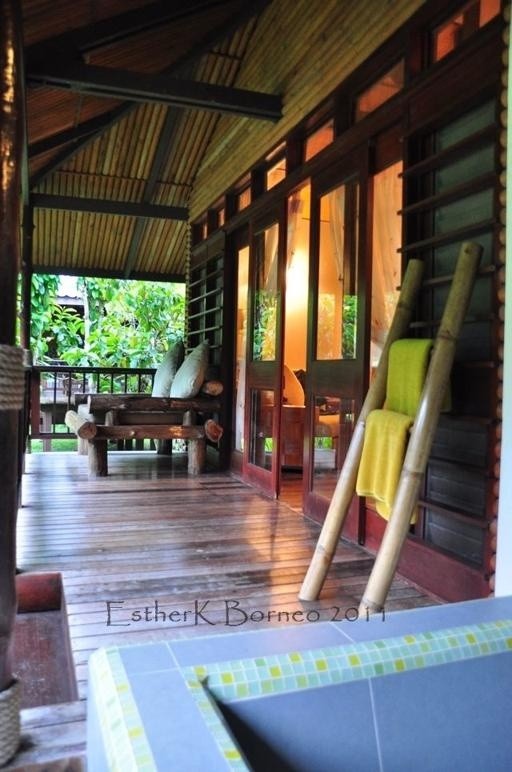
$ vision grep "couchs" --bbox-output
[260,364,340,470]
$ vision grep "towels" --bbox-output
[354,408,420,525]
[386,337,453,419]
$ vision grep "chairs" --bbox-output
[64,377,222,476]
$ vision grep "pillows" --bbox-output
[169,339,210,398]
[150,339,185,398]
